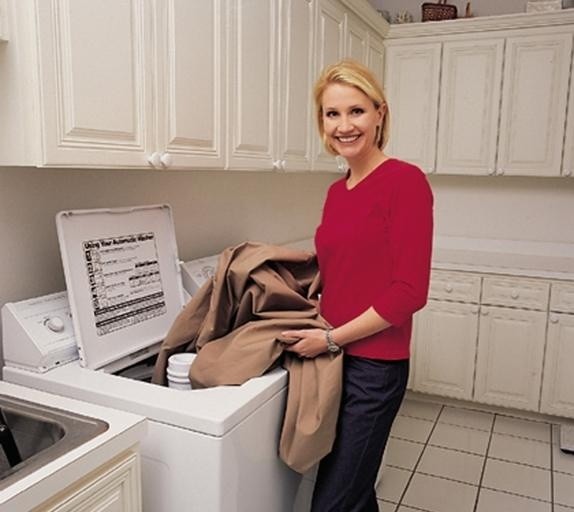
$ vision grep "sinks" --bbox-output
[0,392,108,493]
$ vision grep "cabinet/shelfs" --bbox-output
[313,0,386,172]
[384,16,505,176]
[412,249,550,422]
[19,0,227,170]
[539,266,574,421]
[227,0,311,168]
[498,10,574,174]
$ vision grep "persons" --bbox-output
[283,60,434,511]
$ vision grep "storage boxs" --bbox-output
[423,4,455,19]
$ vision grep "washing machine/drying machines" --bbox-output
[178,239,384,490]
[0,201,305,512]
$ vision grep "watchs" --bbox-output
[325,329,340,353]
